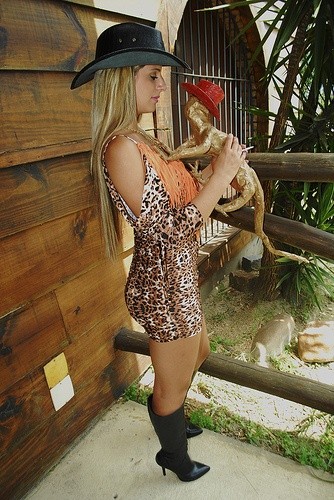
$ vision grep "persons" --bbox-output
[87,24,248,484]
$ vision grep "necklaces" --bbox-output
[124,127,162,149]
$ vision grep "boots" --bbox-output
[183,370,203,438]
[147,394,210,482]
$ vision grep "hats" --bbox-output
[70,22,191,90]
[178,79,224,121]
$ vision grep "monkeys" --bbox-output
[161,92,309,263]
